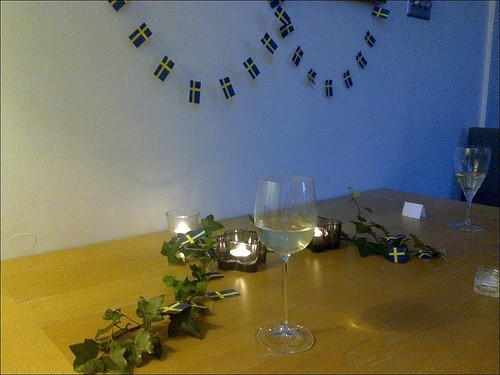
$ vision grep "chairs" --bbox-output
[460,127,500,208]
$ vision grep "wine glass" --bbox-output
[449,146,491,233]
[254,174,319,355]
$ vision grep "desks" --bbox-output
[0,189,500,375]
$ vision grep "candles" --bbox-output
[314,226,328,241]
[174,221,192,236]
[229,242,251,260]
